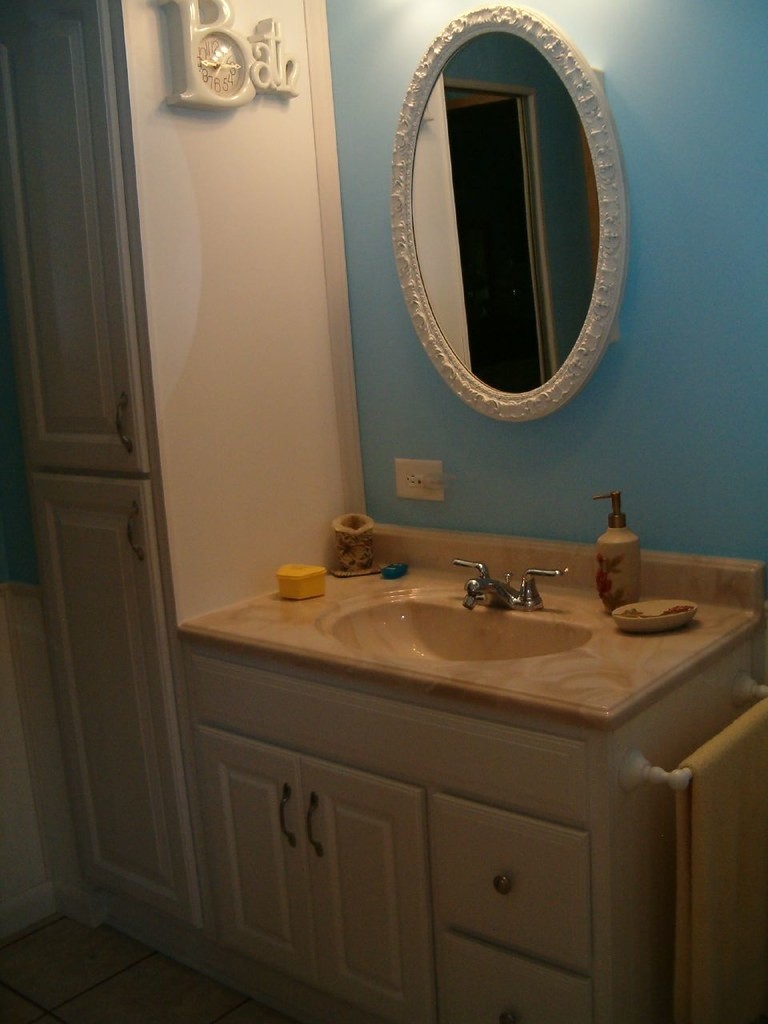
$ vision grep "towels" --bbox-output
[670,694,767,1024]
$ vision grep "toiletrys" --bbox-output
[591,489,644,616]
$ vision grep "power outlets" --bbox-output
[393,458,444,501]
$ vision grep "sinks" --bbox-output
[312,587,598,665]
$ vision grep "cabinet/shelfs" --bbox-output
[0,0,367,971]
[184,641,751,1024]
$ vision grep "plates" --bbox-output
[612,600,698,631]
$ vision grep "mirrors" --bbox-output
[390,1,630,422]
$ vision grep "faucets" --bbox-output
[461,570,518,610]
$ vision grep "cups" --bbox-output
[331,514,376,572]
